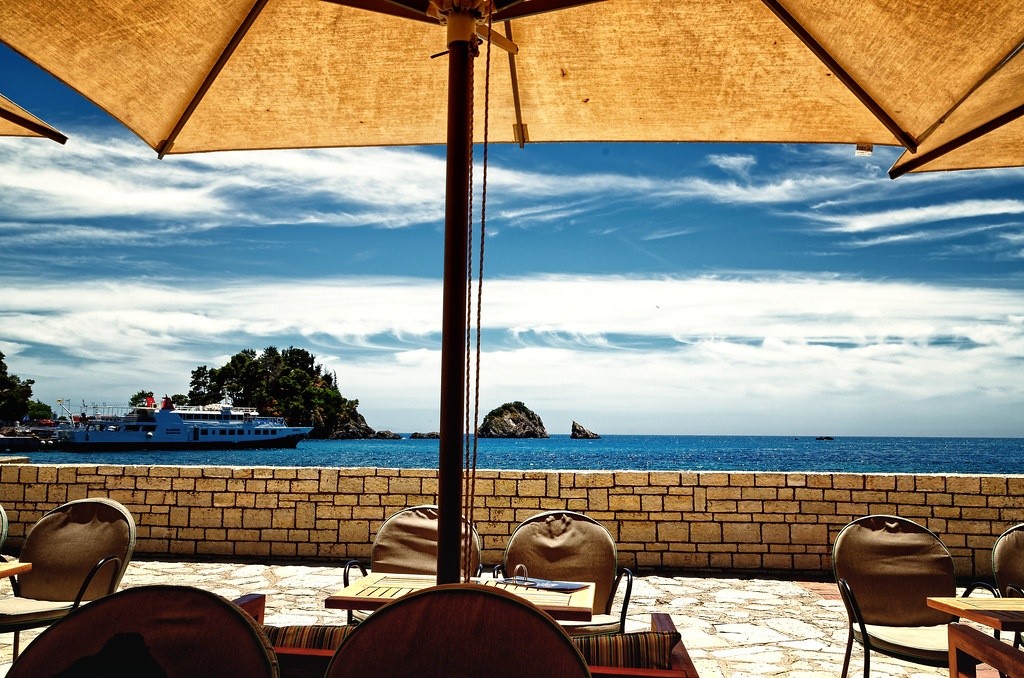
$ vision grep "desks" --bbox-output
[327,571,599,622]
[927,597,1024,678]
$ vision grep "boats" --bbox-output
[58,386,313,449]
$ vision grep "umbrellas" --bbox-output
[0,0,1024,588]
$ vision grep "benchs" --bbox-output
[232,593,702,678]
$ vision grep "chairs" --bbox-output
[831,514,1008,678]
[0,497,136,665]
[344,504,483,626]
[4,584,281,678]
[493,510,633,635]
[323,583,596,678]
[991,522,1024,678]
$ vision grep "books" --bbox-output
[495,576,590,594]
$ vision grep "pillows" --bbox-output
[257,622,356,651]
[568,630,682,668]
